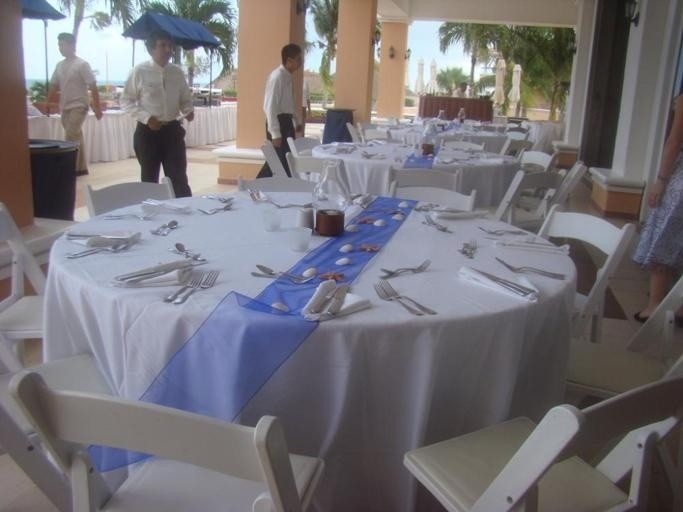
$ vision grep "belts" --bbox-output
[160,120,178,126]
[277,113,294,118]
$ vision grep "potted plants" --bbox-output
[30,82,52,118]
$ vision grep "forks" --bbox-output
[496,258,565,280]
[389,259,433,277]
[424,214,452,233]
[380,279,437,315]
[477,226,528,237]
[374,283,422,317]
[251,272,316,285]
[165,269,206,302]
[66,244,131,260]
[174,269,221,304]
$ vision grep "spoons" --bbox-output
[422,221,448,232]
[163,220,179,236]
[381,267,415,274]
[175,243,207,262]
[256,264,307,279]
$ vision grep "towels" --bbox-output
[435,206,489,220]
[494,233,572,256]
[64,228,141,248]
[342,191,372,204]
[301,279,374,323]
[110,262,193,289]
[458,263,541,304]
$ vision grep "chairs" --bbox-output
[8,367,324,512]
[402,376,683,512]
[390,179,477,212]
[0,203,48,363]
[518,147,560,171]
[559,280,683,403]
[357,122,377,143]
[383,165,464,198]
[513,160,587,239]
[260,138,288,175]
[538,205,636,341]
[438,139,487,152]
[498,138,533,154]
[238,175,323,191]
[283,151,351,197]
[286,135,322,179]
[497,168,563,229]
[345,122,358,141]
[80,179,174,216]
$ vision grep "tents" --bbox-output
[121,11,221,108]
[21,1,67,117]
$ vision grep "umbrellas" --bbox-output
[508,65,522,117]
[415,57,425,95]
[494,59,505,115]
[429,58,437,95]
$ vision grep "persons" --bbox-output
[255,44,302,178]
[46,33,104,176]
[631,46,683,328]
[120,30,194,199]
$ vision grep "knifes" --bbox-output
[119,261,206,283]
[67,234,132,239]
[471,267,534,296]
[327,286,348,318]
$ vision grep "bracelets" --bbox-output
[658,173,668,182]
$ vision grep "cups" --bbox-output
[289,226,312,253]
[261,209,283,232]
[316,210,345,238]
[421,144,434,155]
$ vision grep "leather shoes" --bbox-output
[633,311,651,323]
